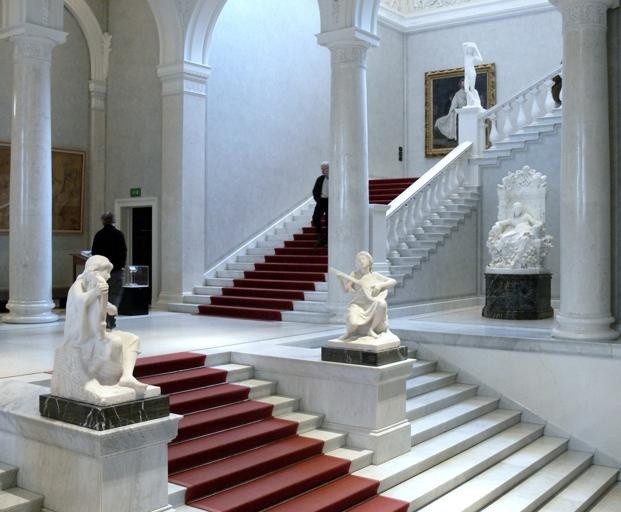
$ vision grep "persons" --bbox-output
[463,43,482,105]
[312,160,329,247]
[435,79,466,141]
[495,201,540,264]
[65,253,149,391]
[337,251,396,340]
[92,212,128,329]
[551,75,562,107]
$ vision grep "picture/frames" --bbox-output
[0,141,87,235]
[425,64,496,157]
